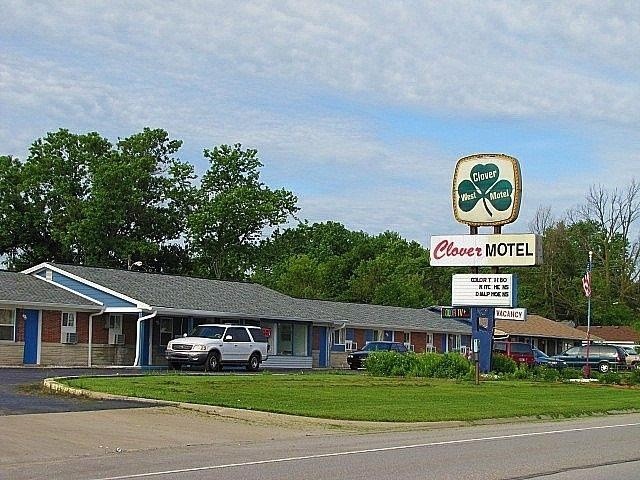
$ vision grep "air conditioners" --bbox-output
[345,340,357,352]
[62,332,78,344]
[426,345,437,352]
[109,333,126,345]
[460,346,470,354]
[404,342,414,352]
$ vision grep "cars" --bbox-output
[532,349,549,367]
[348,341,409,370]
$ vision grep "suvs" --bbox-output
[469,342,534,371]
[165,324,269,371]
[549,345,627,372]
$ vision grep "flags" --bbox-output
[582,263,592,297]
